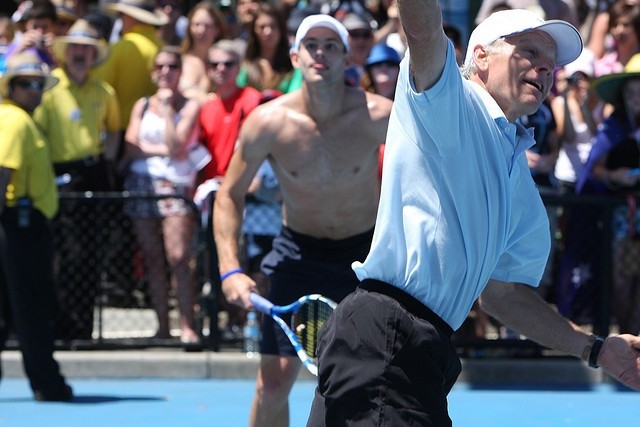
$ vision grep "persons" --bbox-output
[11,0,59,65]
[96,0,160,129]
[185,42,279,188]
[237,8,295,93]
[177,0,228,105]
[365,40,400,98]
[307,0,640,426]
[31,19,121,340]
[343,13,373,90]
[1,51,74,402]
[212,13,394,427]
[576,54,640,337]
[118,49,207,350]
[233,0,263,42]
[516,98,560,302]
[609,0,640,73]
[552,45,614,323]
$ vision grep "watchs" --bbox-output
[587,338,604,369]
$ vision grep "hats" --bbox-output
[295,14,350,54]
[564,48,594,79]
[464,8,583,66]
[106,0,169,26]
[342,13,372,35]
[363,43,400,68]
[54,19,107,63]
[591,53,640,103]
[0,50,59,98]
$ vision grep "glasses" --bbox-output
[153,63,179,69]
[207,60,238,67]
[13,79,44,91]
